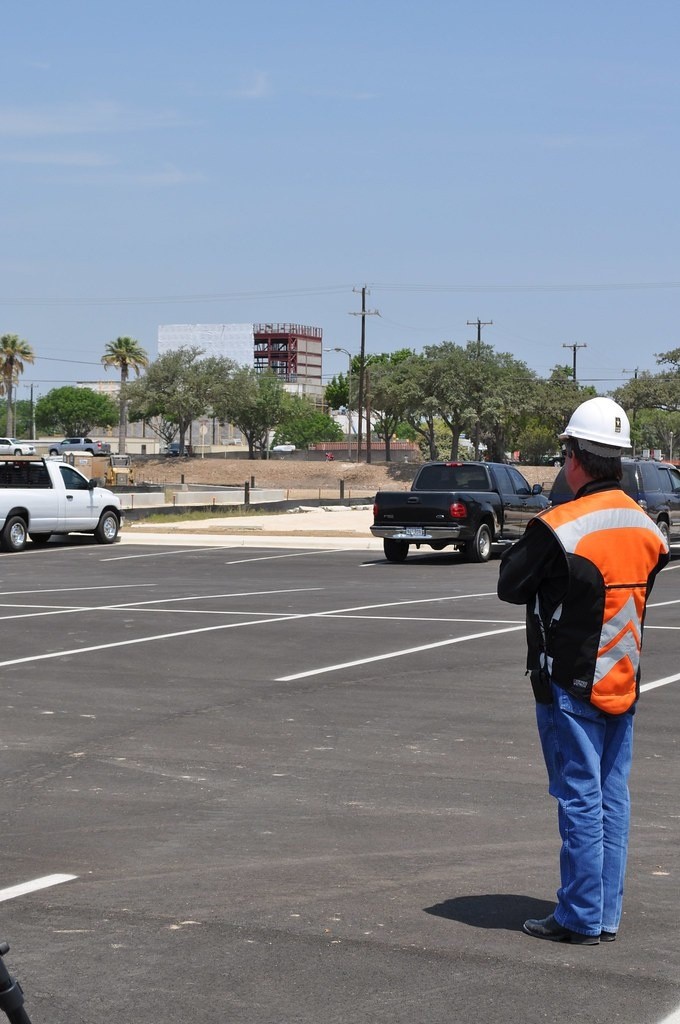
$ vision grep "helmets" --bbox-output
[559,397,633,448]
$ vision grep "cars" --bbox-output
[164,443,190,460]
[0,437,36,457]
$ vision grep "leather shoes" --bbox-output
[523,913,617,945]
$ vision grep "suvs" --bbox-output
[548,456,680,561]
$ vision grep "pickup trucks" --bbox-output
[0,456,123,552]
[369,461,553,562]
[47,436,112,457]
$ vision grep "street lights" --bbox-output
[325,347,352,461]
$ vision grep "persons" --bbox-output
[497,397,671,946]
